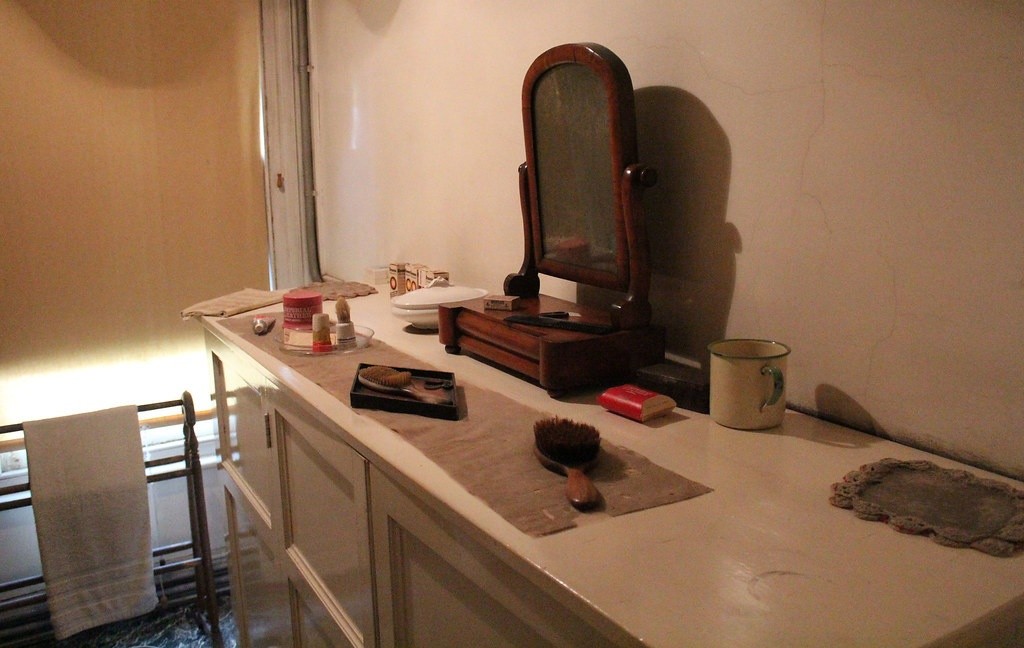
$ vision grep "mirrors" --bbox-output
[439,42,665,398]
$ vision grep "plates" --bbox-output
[275,323,375,355]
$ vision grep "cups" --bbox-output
[708,338,792,430]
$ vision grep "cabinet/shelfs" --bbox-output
[191,282,1024,648]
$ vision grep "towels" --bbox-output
[21,404,160,641]
[179,287,290,321]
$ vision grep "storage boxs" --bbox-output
[388,263,410,298]
[411,265,430,291]
[419,268,449,287]
[404,263,422,292]
